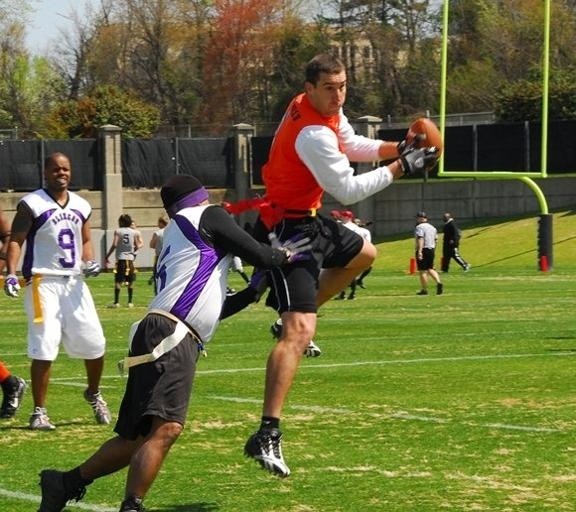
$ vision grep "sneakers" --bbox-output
[333,291,357,301]
[243,429,291,479]
[108,302,120,308]
[119,496,146,512]
[226,288,237,297]
[416,289,428,295]
[436,283,443,295]
[272,318,322,358]
[463,263,471,273]
[36,467,67,512]
[354,279,366,290]
[127,302,135,308]
[28,407,57,431]
[0,376,26,419]
[83,389,112,425]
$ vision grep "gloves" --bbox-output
[83,260,102,278]
[3,273,22,299]
[395,131,442,180]
[268,230,313,266]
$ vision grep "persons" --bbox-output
[438,213,471,273]
[414,211,443,294]
[38,173,314,512]
[244,53,438,477]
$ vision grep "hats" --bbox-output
[159,174,210,218]
[329,210,361,226]
[413,211,429,218]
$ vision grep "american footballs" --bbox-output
[408,117,443,170]
